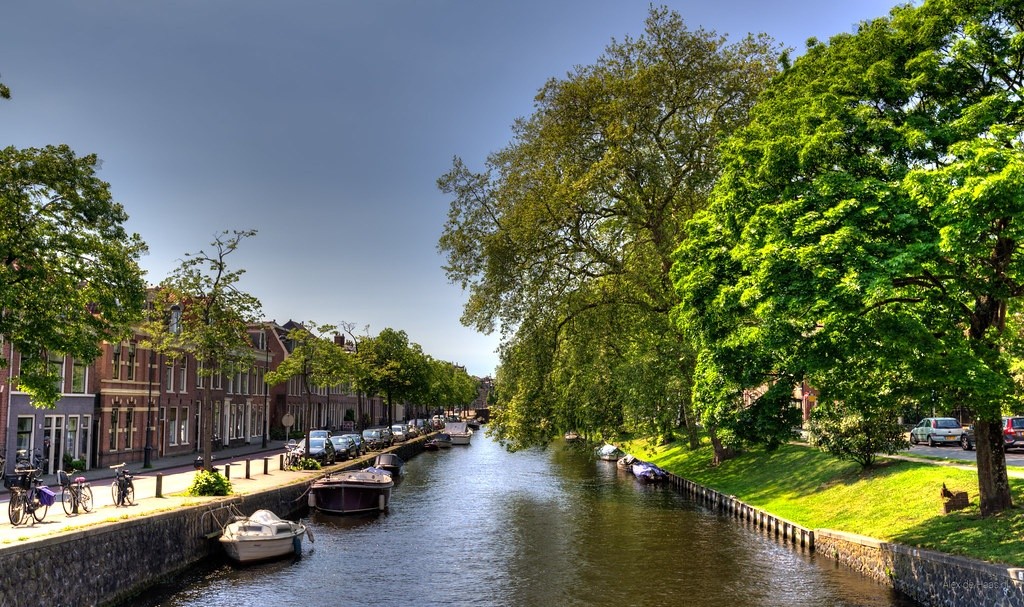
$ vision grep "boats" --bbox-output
[423,440,439,450]
[431,433,454,449]
[373,453,404,476]
[309,467,395,515]
[630,461,668,485]
[218,508,314,569]
[442,423,473,445]
[565,431,578,442]
[597,444,619,460]
[466,419,480,430]
[617,454,641,472]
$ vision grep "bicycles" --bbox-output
[0,450,8,480]
[109,463,136,506]
[283,446,305,471]
[57,469,93,515]
[15,448,44,477]
[4,470,49,526]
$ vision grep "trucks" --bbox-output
[433,415,445,428]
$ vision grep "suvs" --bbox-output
[960,415,1024,450]
[297,430,394,465]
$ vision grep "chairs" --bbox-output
[943,422,948,425]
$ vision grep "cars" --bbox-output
[407,419,434,434]
[910,417,965,447]
[385,425,420,441]
[446,416,461,422]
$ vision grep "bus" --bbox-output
[454,408,467,417]
